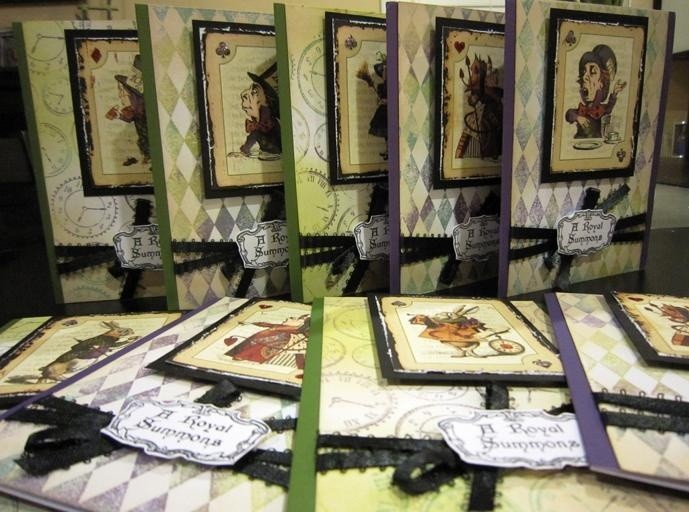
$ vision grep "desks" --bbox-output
[644,228,688,298]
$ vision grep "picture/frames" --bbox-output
[0,311,180,409]
[191,20,285,198]
[146,296,312,402]
[366,293,566,388]
[537,8,649,183]
[324,11,387,185]
[671,122,686,157]
[601,290,688,370]
[64,29,154,196]
[434,16,506,189]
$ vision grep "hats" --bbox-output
[114,54,146,96]
[248,61,279,95]
[579,44,617,102]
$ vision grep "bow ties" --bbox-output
[576,102,604,119]
[245,116,274,134]
[122,100,145,120]
